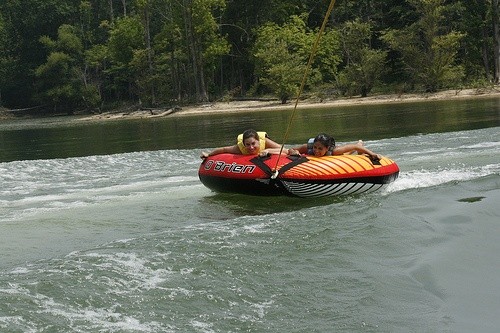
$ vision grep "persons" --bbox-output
[200,129,289,157]
[288,134,382,161]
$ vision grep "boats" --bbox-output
[198,151,400,198]
